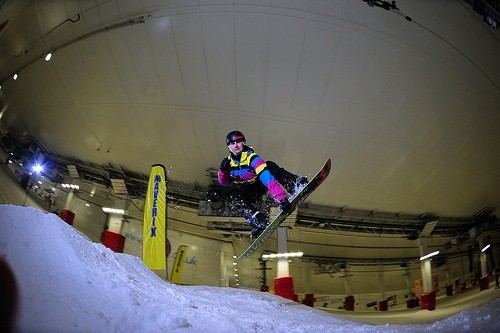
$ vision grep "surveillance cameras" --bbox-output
[106,148,110,153]
[96,146,100,151]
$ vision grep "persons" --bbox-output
[218,130,310,240]
[491,264,500,289]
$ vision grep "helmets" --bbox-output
[226,131,246,145]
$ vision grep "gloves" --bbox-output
[279,198,291,212]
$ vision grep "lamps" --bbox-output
[419,250,440,261]
[102,205,128,216]
[262,251,305,260]
[480,244,490,253]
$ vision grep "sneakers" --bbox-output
[294,176,309,194]
[248,213,267,239]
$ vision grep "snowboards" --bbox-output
[235,158,331,263]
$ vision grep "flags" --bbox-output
[171,244,188,283]
[142,164,168,270]
